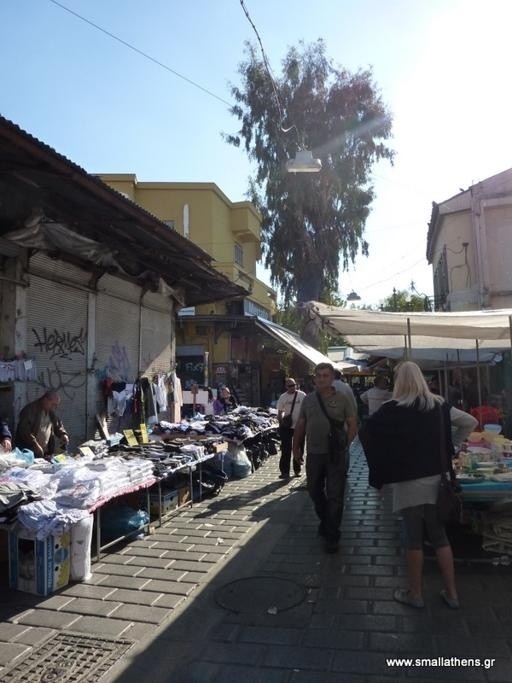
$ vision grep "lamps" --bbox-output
[278,112,322,173]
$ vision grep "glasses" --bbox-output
[286,384,296,388]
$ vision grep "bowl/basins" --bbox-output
[458,447,512,484]
[484,424,502,435]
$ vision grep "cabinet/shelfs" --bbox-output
[0,407,283,563]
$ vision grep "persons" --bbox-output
[292,364,359,556]
[213,352,487,480]
[14,390,70,460]
[0,411,13,453]
[359,360,479,612]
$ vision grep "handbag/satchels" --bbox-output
[278,391,299,430]
[316,392,348,450]
[425,400,463,533]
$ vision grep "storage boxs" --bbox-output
[8,519,71,598]
[138,490,178,515]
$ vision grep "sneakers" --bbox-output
[441,590,460,609]
[318,522,340,554]
[391,589,424,608]
[279,473,302,478]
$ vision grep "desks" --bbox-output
[424,432,512,525]
